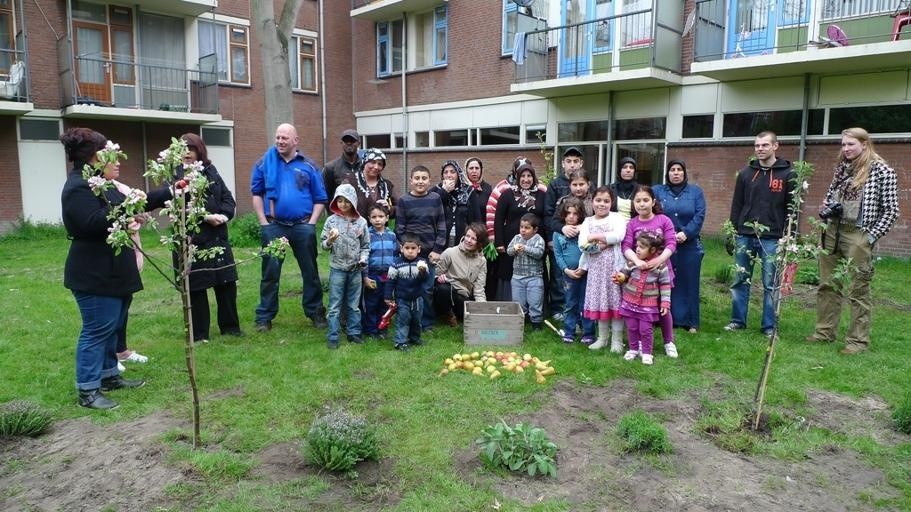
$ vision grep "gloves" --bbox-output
[726,238,734,256]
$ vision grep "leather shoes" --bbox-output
[98,374,144,392]
[78,389,119,409]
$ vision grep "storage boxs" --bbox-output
[463,301,525,345]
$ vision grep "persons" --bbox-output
[486,147,597,341]
[724,131,798,340]
[59,127,188,410]
[105,154,148,372]
[321,130,493,351]
[251,123,329,332]
[162,133,246,343]
[806,127,899,355]
[578,156,705,366]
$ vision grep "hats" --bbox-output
[341,129,360,141]
[563,148,584,157]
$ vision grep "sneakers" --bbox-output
[841,348,856,354]
[766,331,776,336]
[117,350,147,362]
[252,322,423,349]
[724,323,741,330]
[806,336,818,341]
[117,361,127,374]
[535,316,681,365]
